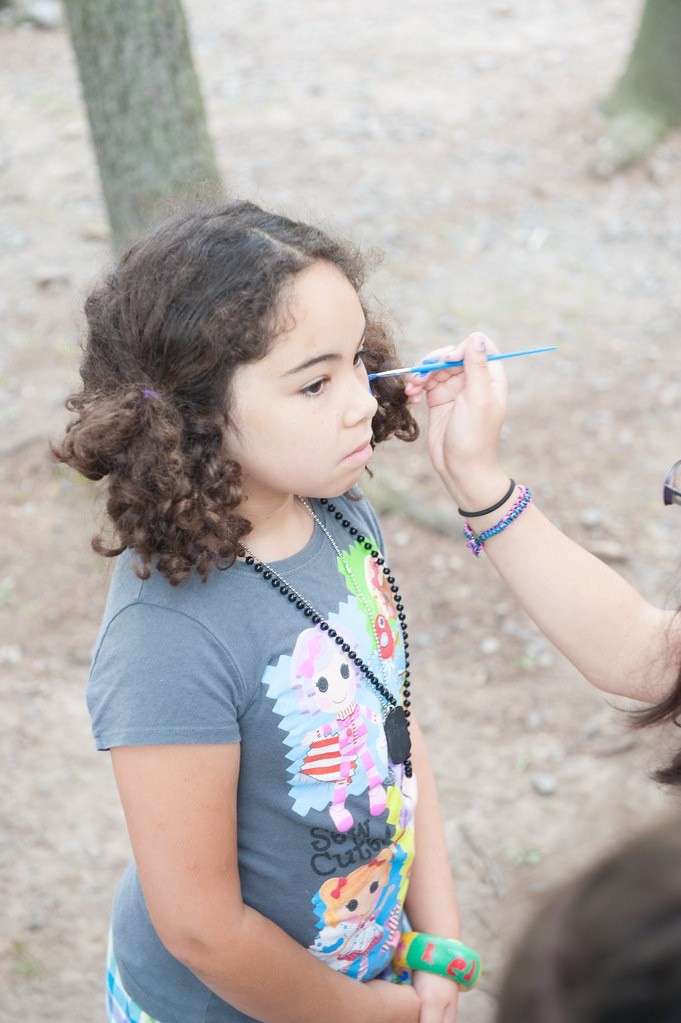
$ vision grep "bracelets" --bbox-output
[456,479,515,516]
[462,485,531,556]
[392,930,482,993]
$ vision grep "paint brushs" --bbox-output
[367,345,558,381]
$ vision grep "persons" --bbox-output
[45,196,481,1023]
[404,334,680,786]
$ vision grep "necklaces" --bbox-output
[235,493,414,785]
[229,491,394,725]
